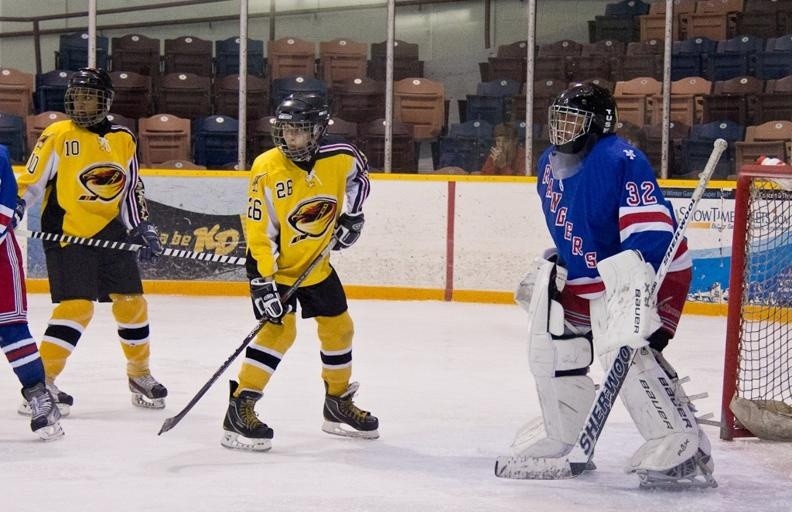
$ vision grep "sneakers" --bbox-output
[128,374,168,399]
[508,427,594,458]
[223,379,273,439]
[21,379,61,432]
[44,383,73,406]
[638,427,711,476]
[323,380,378,431]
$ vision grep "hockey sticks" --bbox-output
[495,138,727,479]
[158,237,337,434]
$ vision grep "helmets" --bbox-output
[64,68,116,128]
[272,92,332,161]
[547,83,619,162]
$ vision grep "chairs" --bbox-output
[735,141,788,177]
[670,35,718,83]
[139,114,192,166]
[271,77,329,116]
[497,40,539,57]
[754,91,792,124]
[713,74,765,94]
[745,120,792,167]
[649,1,696,14]
[370,39,419,79]
[765,74,792,92]
[331,76,386,121]
[690,119,746,148]
[638,137,675,179]
[617,120,647,153]
[466,94,505,126]
[476,78,521,96]
[671,76,713,122]
[164,36,213,76]
[35,69,75,114]
[450,119,495,147]
[761,33,792,77]
[716,33,765,79]
[687,12,729,40]
[696,1,744,12]
[367,127,417,175]
[158,72,211,116]
[487,56,524,82]
[583,34,640,80]
[511,97,549,125]
[25,111,69,162]
[613,94,646,129]
[745,0,790,15]
[267,36,316,79]
[320,38,367,82]
[681,139,731,180]
[150,159,207,170]
[703,94,746,123]
[624,39,665,81]
[55,31,109,71]
[0,112,24,163]
[534,55,566,79]
[393,77,444,140]
[595,15,640,42]
[215,36,264,74]
[215,74,266,118]
[735,9,790,38]
[107,70,152,119]
[540,39,583,81]
[522,78,568,97]
[639,13,679,42]
[615,77,663,122]
[191,114,239,169]
[111,34,160,73]
[0,68,33,119]
[651,95,695,126]
[604,0,650,15]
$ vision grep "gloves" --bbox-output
[249,278,292,326]
[12,197,26,228]
[646,327,673,351]
[130,222,162,264]
[330,213,365,250]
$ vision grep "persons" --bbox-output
[495,82,716,488]
[216,93,379,451]
[15,67,170,417]
[0,143,65,443]
[482,123,539,177]
